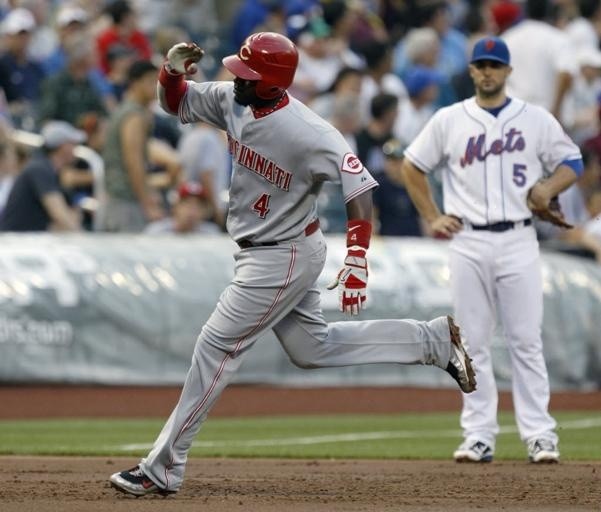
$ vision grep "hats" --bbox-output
[406,66,450,98]
[469,35,511,66]
[1,6,91,149]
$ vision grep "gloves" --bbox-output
[163,41,204,76]
[326,245,370,317]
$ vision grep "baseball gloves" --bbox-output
[527,176,573,228]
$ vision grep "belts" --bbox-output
[472,219,532,232]
[235,240,277,249]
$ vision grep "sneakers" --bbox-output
[452,440,493,463]
[530,449,560,464]
[445,314,478,394]
[108,465,178,498]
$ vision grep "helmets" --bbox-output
[221,31,300,100]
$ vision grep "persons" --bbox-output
[400,37,585,464]
[110,32,477,497]
